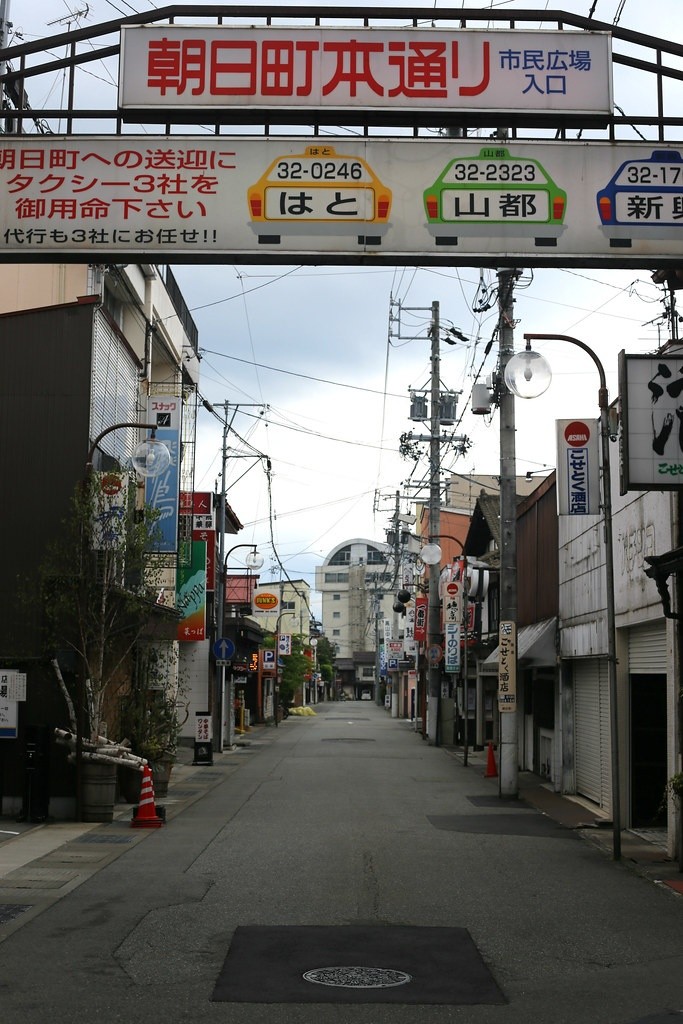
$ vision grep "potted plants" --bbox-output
[118,694,177,803]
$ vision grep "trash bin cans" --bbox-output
[277,705,284,721]
[16,723,48,822]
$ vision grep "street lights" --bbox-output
[82,421,171,738]
[223,543,264,637]
[421,533,469,768]
[505,333,622,863]
[274,613,298,727]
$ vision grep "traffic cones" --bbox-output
[485,742,498,777]
[132,765,164,829]
